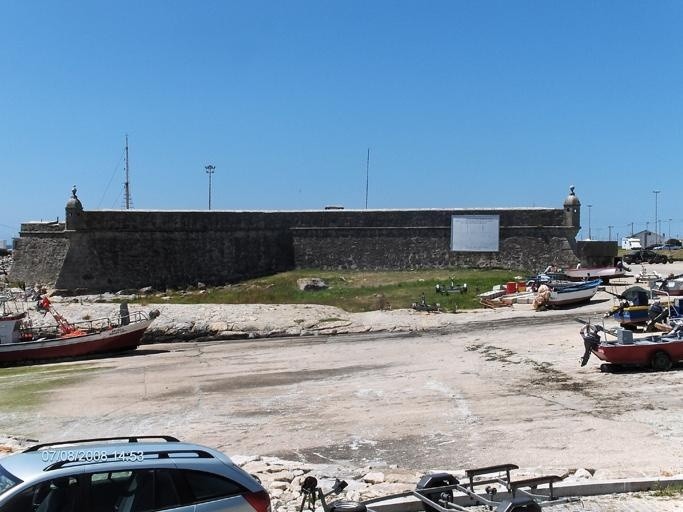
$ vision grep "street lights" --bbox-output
[206,165,216,211]
[608,225,613,240]
[586,204,592,241]
[667,219,672,238]
[653,189,661,243]
[630,222,634,237]
[615,232,619,241]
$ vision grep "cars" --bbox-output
[652,243,682,251]
[622,249,668,265]
[0,435,271,512]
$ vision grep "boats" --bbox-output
[584,273,683,372]
[1,264,160,364]
[531,273,603,309]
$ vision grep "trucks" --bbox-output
[622,237,643,250]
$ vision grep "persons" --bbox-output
[543,264,554,274]
[586,273,590,281]
[421,292,425,304]
[575,260,581,270]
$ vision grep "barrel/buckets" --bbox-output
[516,281,526,292]
[506,282,517,293]
